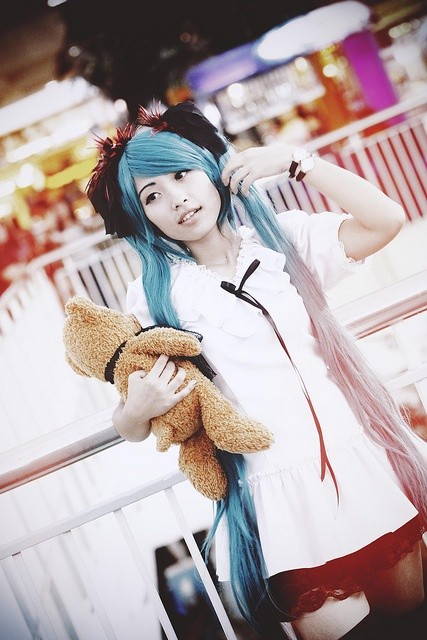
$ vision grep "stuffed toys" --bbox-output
[63,294,274,504]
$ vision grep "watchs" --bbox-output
[296,151,317,183]
[288,146,308,179]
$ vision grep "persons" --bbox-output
[63,98,427,640]
